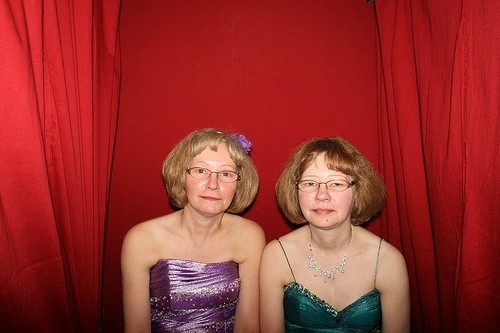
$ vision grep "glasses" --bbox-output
[295,179,355,191]
[186,166,241,182]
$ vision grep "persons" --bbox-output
[259,137,411,333]
[120,128,266,333]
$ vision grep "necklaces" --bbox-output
[307,225,353,283]
